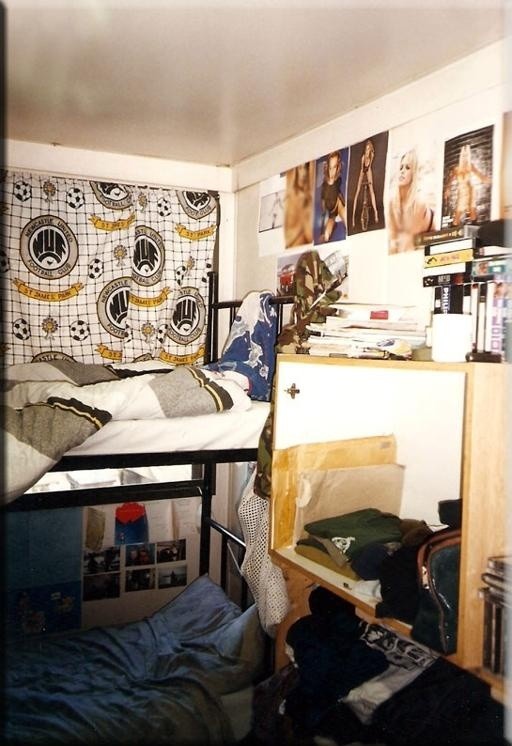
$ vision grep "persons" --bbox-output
[318,151,347,242]
[444,141,491,228]
[351,138,381,229]
[389,147,437,254]
[283,160,314,246]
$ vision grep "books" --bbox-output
[493,606,503,673]
[477,586,512,608]
[482,598,496,671]
[484,553,512,573]
[479,571,512,593]
[304,231,512,363]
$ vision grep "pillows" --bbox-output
[155,573,266,699]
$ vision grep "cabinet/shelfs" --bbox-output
[268,353,512,704]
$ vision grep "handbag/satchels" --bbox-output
[410,527,461,657]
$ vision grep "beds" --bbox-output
[1,251,320,744]
